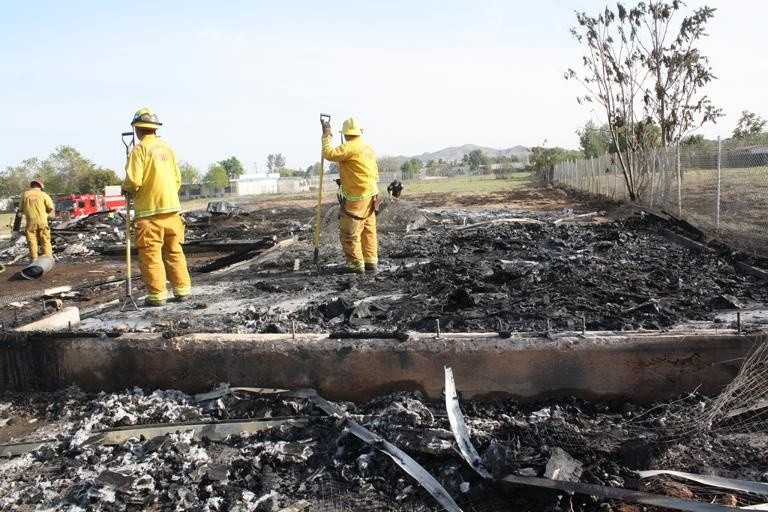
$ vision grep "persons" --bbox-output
[320,116,378,272]
[387,180,403,199]
[19,179,54,263]
[122,107,192,306]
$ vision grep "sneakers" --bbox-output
[172,294,189,302]
[337,263,378,274]
[142,296,166,307]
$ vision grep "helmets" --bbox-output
[341,117,361,137]
[130,107,163,131]
[29,180,45,191]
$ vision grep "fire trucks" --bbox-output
[57,193,126,220]
